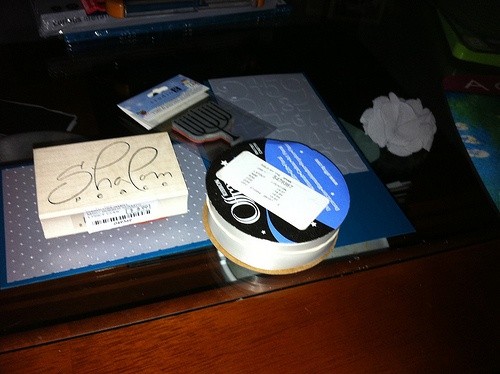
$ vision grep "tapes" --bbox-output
[203,134,349,275]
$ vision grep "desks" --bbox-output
[2,234,499,374]
